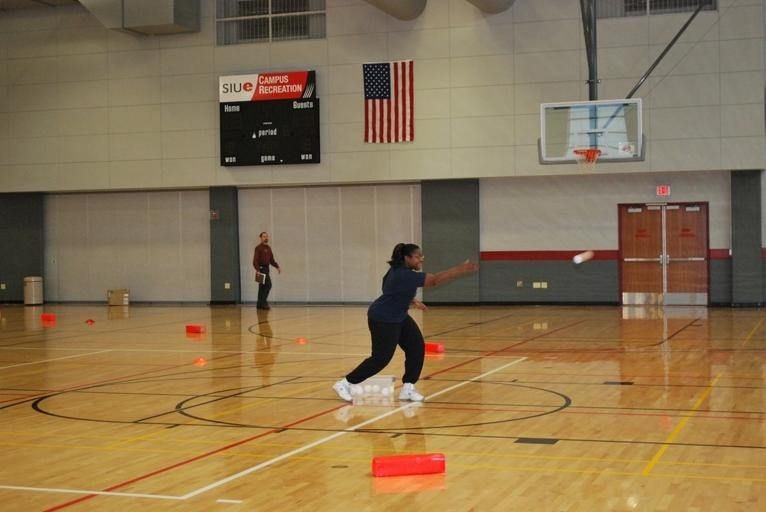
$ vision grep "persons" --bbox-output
[254,232,281,310]
[332,243,480,402]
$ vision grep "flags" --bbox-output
[364,61,414,143]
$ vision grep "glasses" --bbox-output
[411,253,425,259]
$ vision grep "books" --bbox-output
[255,272,266,285]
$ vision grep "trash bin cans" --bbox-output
[24,276,43,305]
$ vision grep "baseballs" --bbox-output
[573,255,584,264]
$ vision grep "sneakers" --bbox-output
[332,380,354,402]
[398,387,425,402]
[261,303,270,310]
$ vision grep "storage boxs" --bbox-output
[107,288,129,306]
